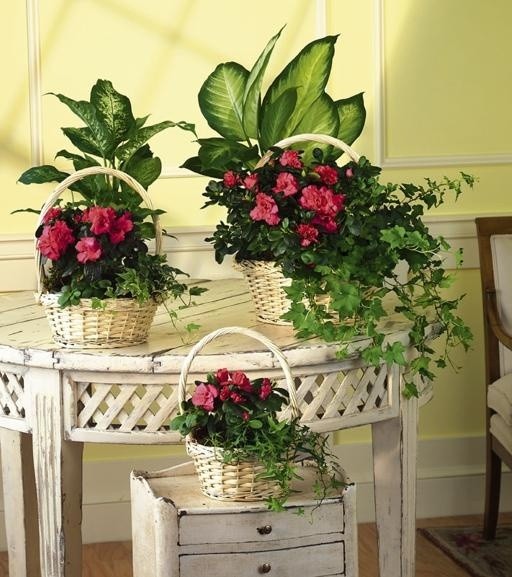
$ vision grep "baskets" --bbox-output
[233,133,362,326]
[179,327,297,502]
[33,166,166,348]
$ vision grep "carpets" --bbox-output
[419,518,512,576]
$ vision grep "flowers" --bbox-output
[174,20,476,400]
[170,368,350,526]
[6,78,213,347]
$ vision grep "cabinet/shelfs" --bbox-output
[129,458,363,576]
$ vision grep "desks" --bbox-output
[1,280,448,576]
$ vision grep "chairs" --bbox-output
[468,215,511,537]
